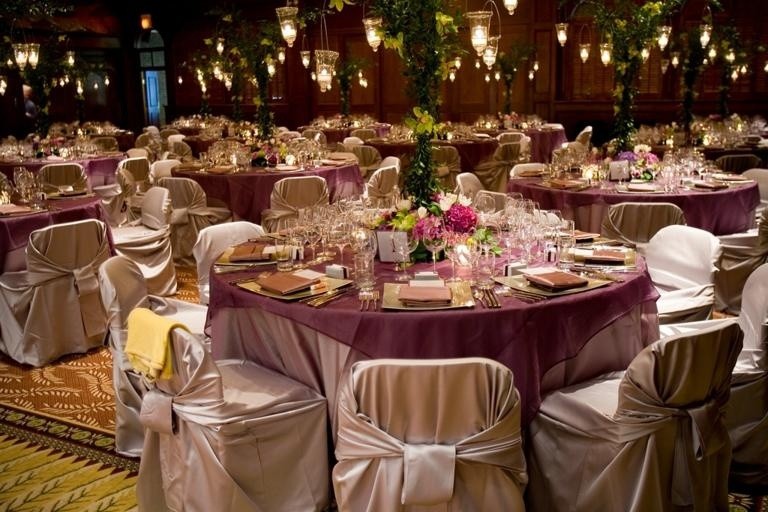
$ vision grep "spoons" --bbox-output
[470,281,500,308]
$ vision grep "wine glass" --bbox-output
[0,166,41,211]
[544,150,713,191]
[278,193,575,282]
[638,116,748,150]
[1,111,543,173]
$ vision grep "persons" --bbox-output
[22,84,37,132]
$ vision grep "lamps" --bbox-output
[0,17,110,99]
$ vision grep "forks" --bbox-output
[358,287,380,311]
